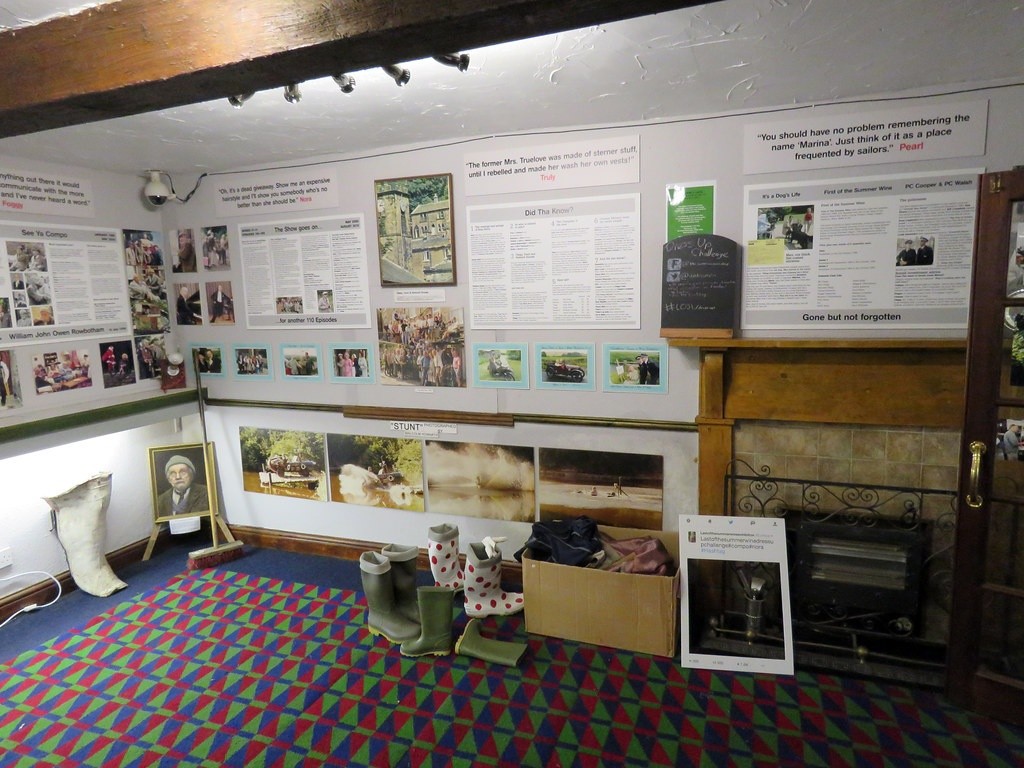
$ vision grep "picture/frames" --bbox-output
[373,172,457,286]
[148,441,219,523]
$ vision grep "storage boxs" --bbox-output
[521,525,680,659]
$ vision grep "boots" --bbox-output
[455,619,529,668]
[360,551,422,644]
[464,542,524,618]
[428,523,465,596]
[400,586,453,657]
[381,543,421,624]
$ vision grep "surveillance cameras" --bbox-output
[145,182,169,205]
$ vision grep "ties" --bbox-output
[174,490,186,515]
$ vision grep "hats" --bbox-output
[920,237,928,242]
[165,455,195,475]
[641,354,648,358]
[905,240,913,244]
[635,356,641,360]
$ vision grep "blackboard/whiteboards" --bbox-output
[662,234,741,336]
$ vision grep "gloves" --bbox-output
[481,536,509,560]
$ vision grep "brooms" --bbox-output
[184,348,246,569]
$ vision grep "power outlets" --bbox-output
[0,545,13,570]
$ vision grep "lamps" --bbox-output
[382,66,411,87]
[283,85,306,104]
[332,74,357,93]
[431,54,470,71]
[228,91,253,108]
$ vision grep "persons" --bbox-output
[635,353,660,385]
[319,292,332,312]
[176,287,202,325]
[1003,421,1024,462]
[177,232,196,271]
[198,349,221,373]
[124,232,162,310]
[210,285,231,323]
[10,242,55,326]
[757,207,817,238]
[305,351,314,375]
[140,337,163,376]
[336,350,368,378]
[100,346,130,376]
[204,230,227,267]
[896,236,933,267]
[34,355,89,389]
[238,352,264,375]
[381,314,465,387]
